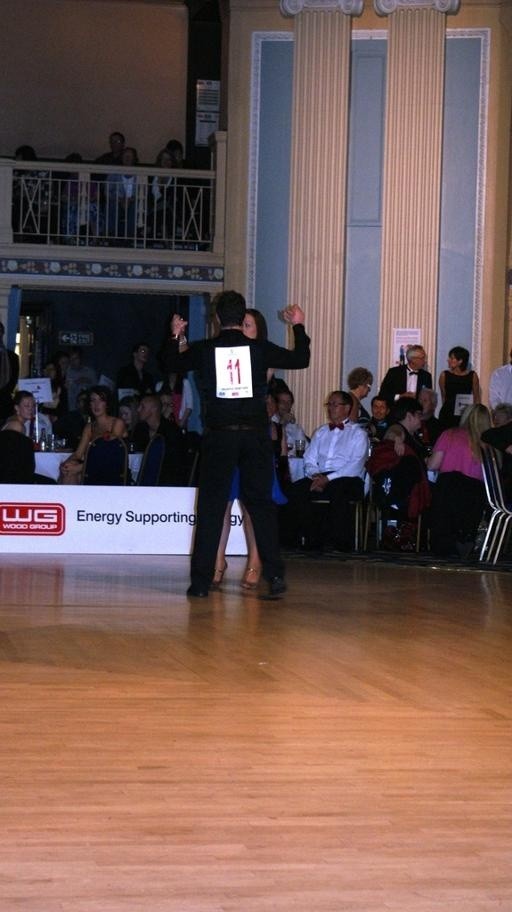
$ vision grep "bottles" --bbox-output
[294,423,306,458]
[49,433,55,450]
[38,427,48,451]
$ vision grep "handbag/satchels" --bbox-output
[383,523,425,552]
[365,440,400,475]
[408,479,432,517]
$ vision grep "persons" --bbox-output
[12,133,207,252]
[170,290,311,596]
[267,346,511,562]
[1,324,65,484]
[52,342,202,487]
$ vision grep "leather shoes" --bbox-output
[263,570,286,592]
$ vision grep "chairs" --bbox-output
[80,434,512,570]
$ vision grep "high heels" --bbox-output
[210,559,227,587]
[240,568,262,589]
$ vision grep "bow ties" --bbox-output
[329,423,344,430]
[409,371,421,375]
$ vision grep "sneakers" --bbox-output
[188,585,208,597]
